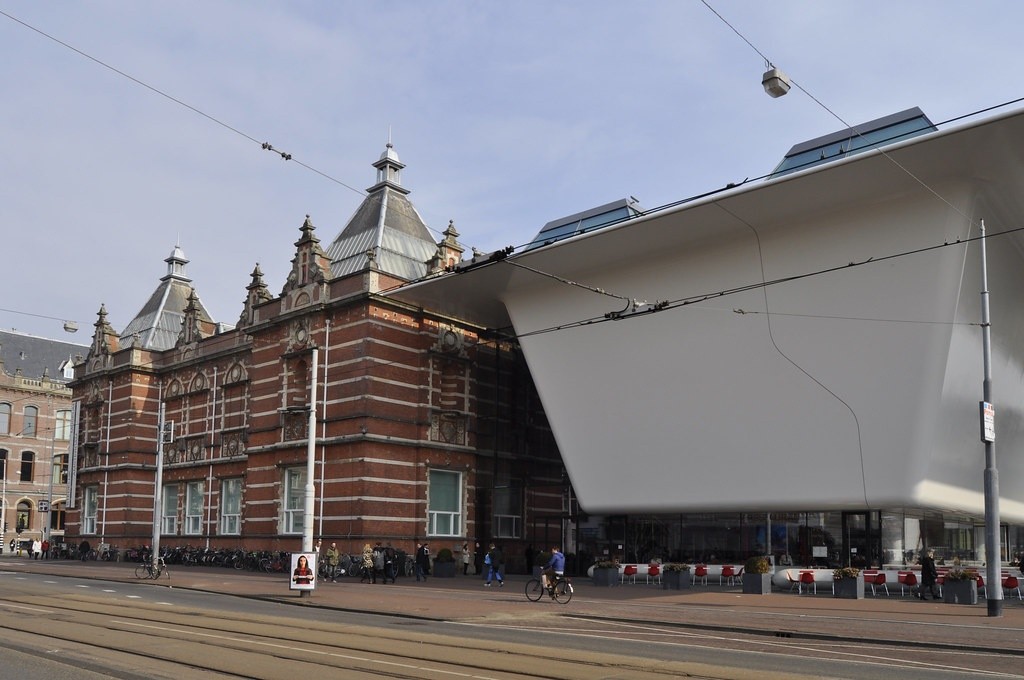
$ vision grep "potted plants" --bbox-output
[942,569,977,605]
[533,552,553,580]
[832,567,865,599]
[432,548,456,578]
[742,557,772,594]
[662,563,691,590]
[593,561,622,588]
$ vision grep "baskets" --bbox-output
[533,566,548,578]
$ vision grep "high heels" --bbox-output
[921,596,928,600]
[933,595,942,600]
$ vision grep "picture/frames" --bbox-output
[289,551,318,591]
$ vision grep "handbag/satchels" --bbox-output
[543,574,552,588]
[484,553,491,564]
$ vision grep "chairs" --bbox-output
[693,564,710,586]
[786,569,1022,601]
[728,567,745,586]
[646,563,660,585]
[719,565,736,586]
[622,565,637,584]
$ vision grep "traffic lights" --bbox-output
[4,522,8,532]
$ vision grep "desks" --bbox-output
[1002,577,1024,600]
[897,574,922,597]
[797,573,816,594]
[863,574,879,594]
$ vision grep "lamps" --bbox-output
[63,321,79,332]
[762,66,791,98]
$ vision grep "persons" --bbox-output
[539,546,565,597]
[361,544,395,584]
[79,541,90,561]
[1014,556,1024,575]
[416,543,431,581]
[474,543,482,575]
[10,539,16,555]
[293,556,314,584]
[313,540,325,576]
[920,550,941,600]
[779,551,793,566]
[462,544,471,575]
[326,542,340,583]
[484,544,504,586]
[27,538,49,560]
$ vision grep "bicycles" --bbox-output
[525,567,574,604]
[50,547,120,561]
[135,554,170,580]
[125,544,416,578]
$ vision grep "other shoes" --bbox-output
[498,582,504,587]
[416,579,420,581]
[369,579,372,583]
[324,577,326,581]
[422,577,426,582]
[333,579,336,583]
[372,580,376,584]
[392,579,395,583]
[383,580,387,584]
[483,583,491,586]
[361,579,365,583]
